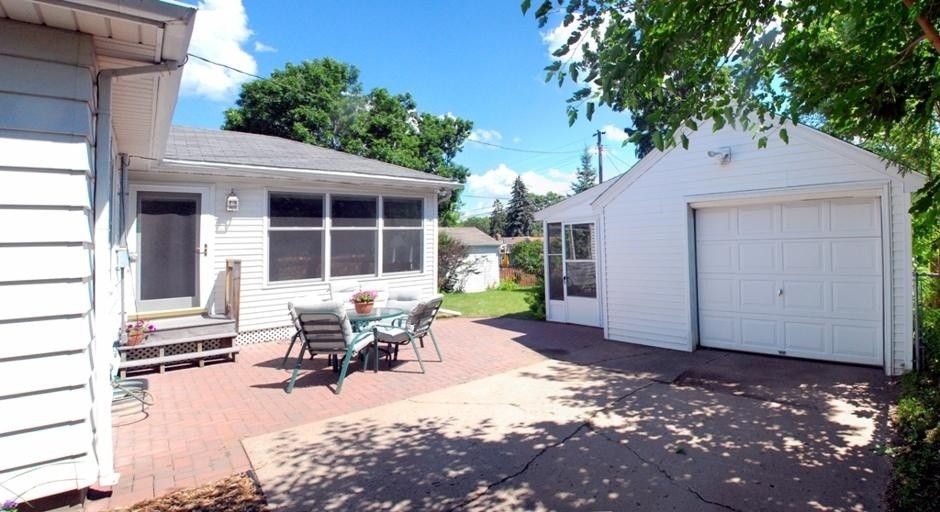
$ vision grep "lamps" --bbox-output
[227,187,239,212]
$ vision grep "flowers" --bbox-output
[350,292,378,302]
[127,320,157,334]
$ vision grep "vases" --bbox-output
[126,337,146,346]
[353,302,374,313]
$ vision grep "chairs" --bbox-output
[387,292,418,327]
[375,295,445,373]
[286,301,375,395]
[278,301,315,372]
